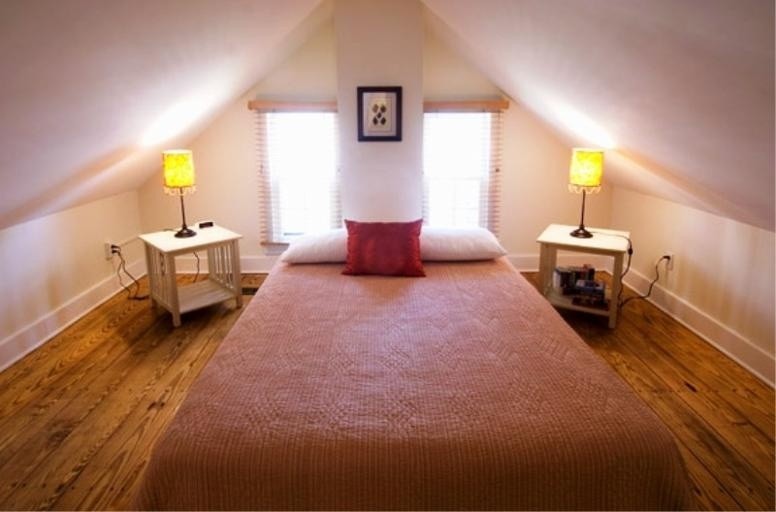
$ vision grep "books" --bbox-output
[553,263,610,311]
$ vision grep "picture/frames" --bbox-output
[357,86,402,142]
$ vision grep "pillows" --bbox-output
[279,217,507,278]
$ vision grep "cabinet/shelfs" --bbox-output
[136,223,243,328]
[533,224,631,329]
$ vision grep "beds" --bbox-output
[130,249,696,512]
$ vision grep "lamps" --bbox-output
[566,148,604,238]
[163,148,197,237]
